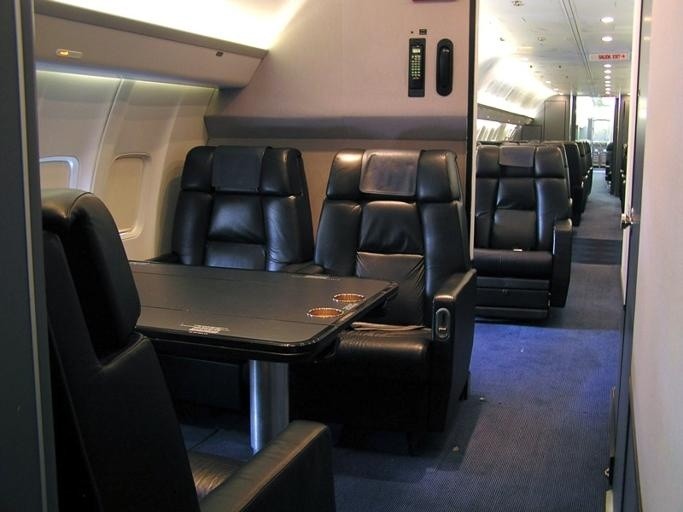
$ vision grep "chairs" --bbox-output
[40,187,336,511]
[146,143,315,420]
[289,147,476,459]
[474,139,593,325]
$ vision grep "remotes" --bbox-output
[410,45,423,88]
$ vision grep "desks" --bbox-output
[127,258,400,455]
